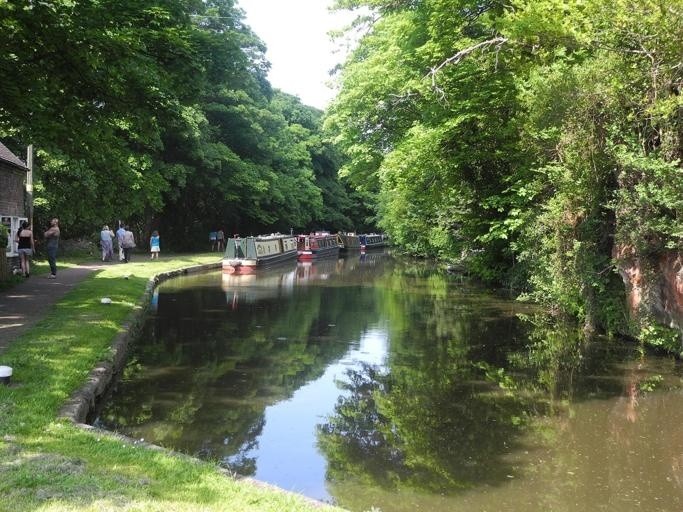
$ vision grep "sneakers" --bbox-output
[47,272,56,280]
[21,271,29,279]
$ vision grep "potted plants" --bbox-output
[0,223,10,249]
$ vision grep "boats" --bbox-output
[221,230,391,273]
[219,252,392,311]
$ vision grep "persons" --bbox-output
[44,218,60,279]
[116,224,126,261]
[150,230,161,260]
[208,228,217,252]
[101,225,115,262]
[15,222,36,278]
[217,228,225,252]
[121,226,135,262]
